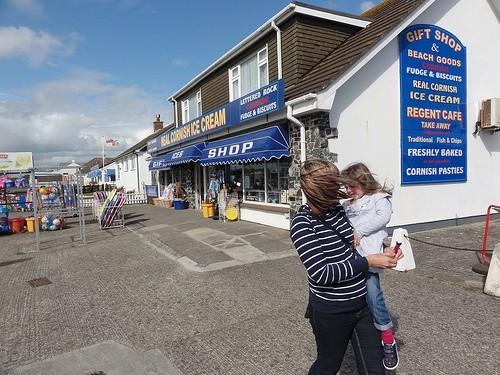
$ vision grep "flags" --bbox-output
[105,138,115,143]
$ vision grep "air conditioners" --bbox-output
[473,97,500,135]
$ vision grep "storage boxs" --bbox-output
[10,218,25,232]
[202,204,214,217]
[173,199,187,209]
[26,216,41,233]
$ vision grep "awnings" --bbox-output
[148,125,292,172]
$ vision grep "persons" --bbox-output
[341,164,400,370]
[209,173,220,211]
[290,160,404,375]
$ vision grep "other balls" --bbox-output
[38,184,68,231]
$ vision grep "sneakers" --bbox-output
[382,337,399,370]
